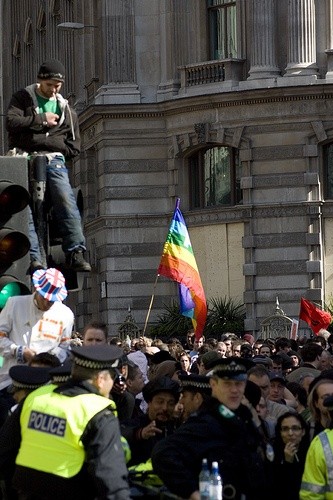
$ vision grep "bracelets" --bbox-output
[16,345,25,364]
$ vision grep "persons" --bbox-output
[0,268,75,414]
[0,323,333,500]
[5,59,92,272]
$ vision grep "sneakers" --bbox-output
[65,250,90,272]
[31,260,43,270]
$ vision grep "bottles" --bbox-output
[198,458,223,500]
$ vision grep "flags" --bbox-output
[298,297,331,335]
[157,200,207,340]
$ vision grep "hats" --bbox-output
[210,357,249,380]
[269,372,284,383]
[32,268,68,302]
[52,366,71,382]
[37,59,65,84]
[70,345,123,369]
[8,365,54,388]
[150,361,181,381]
[142,378,180,404]
[178,375,213,394]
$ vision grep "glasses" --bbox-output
[280,426,303,433]
